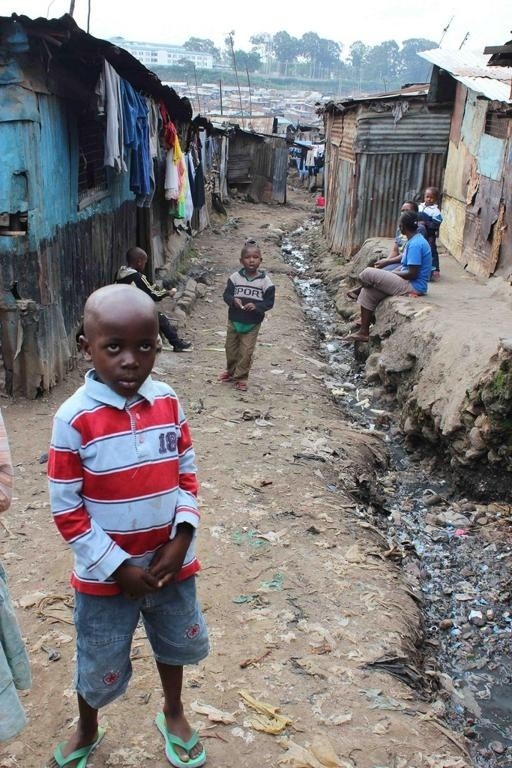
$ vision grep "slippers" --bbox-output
[155,712,206,768]
[53,726,104,768]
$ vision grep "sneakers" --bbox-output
[220,372,248,391]
[173,340,191,351]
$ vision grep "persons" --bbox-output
[112,245,193,354]
[372,200,427,272]
[39,282,210,768]
[417,186,443,279]
[217,244,275,392]
[340,209,433,343]
[2,408,35,747]
